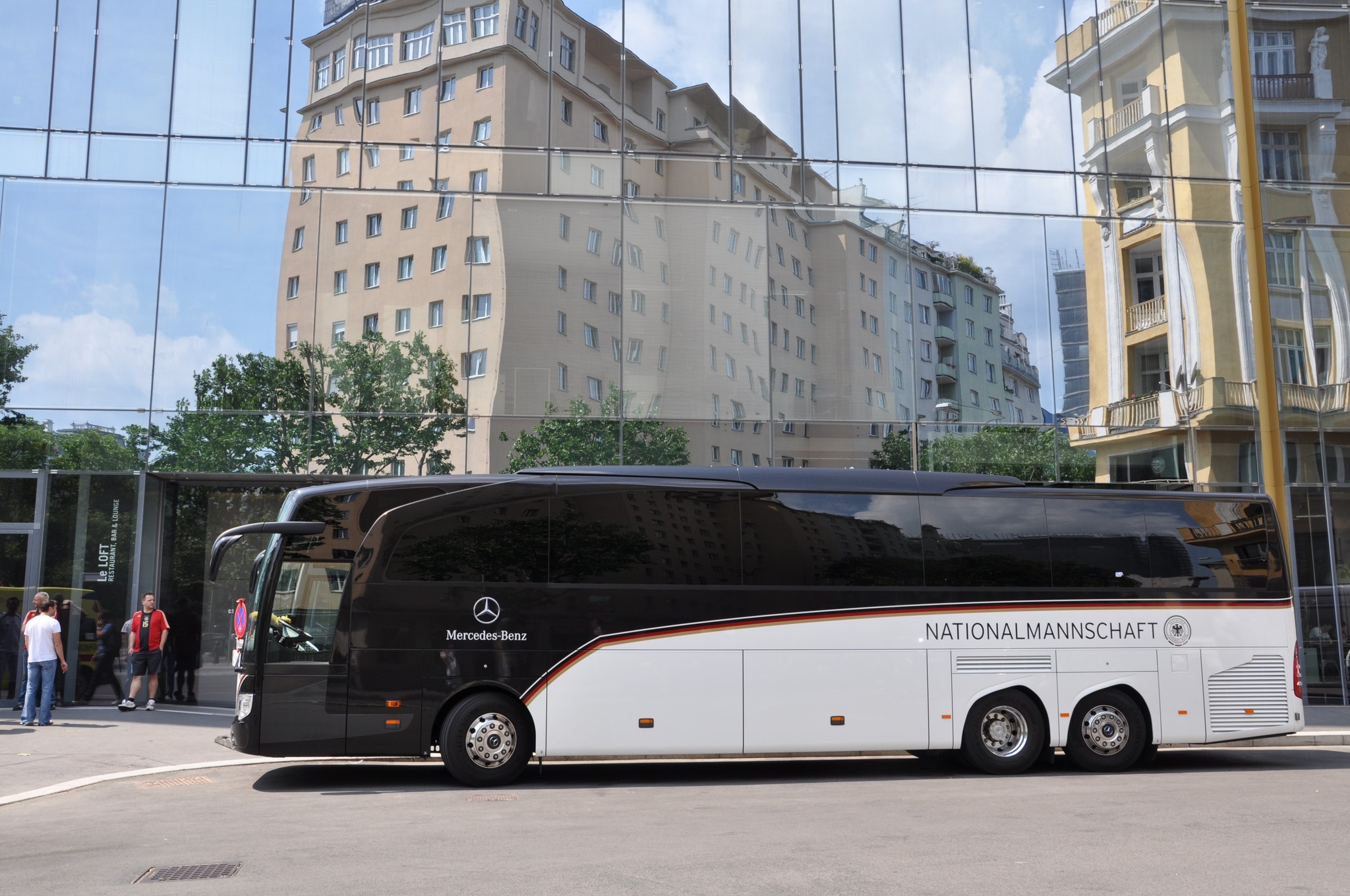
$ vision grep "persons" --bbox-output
[20,599,68,725]
[173,594,201,706]
[11,591,58,711]
[121,617,138,686]
[76,611,126,704]
[1309,621,1348,670]
[0,597,23,701]
[117,593,170,711]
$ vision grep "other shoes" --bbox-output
[19,722,33,726]
[165,695,170,699]
[40,721,54,726]
[111,697,125,704]
[175,691,184,700]
[187,693,197,703]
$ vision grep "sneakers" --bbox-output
[13,704,24,710]
[118,700,136,712]
[145,701,154,711]
[50,705,55,710]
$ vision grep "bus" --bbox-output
[210,465,1304,789]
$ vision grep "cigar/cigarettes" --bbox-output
[63,671,65,673]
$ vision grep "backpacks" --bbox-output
[106,624,122,657]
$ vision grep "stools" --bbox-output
[1318,657,1341,677]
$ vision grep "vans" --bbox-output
[0,586,104,707]
